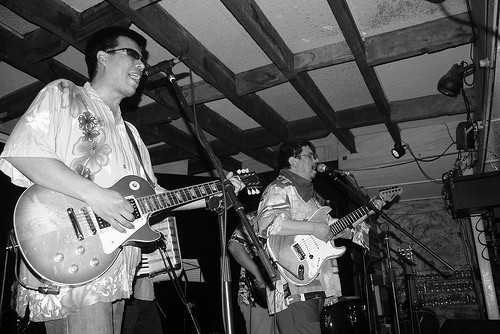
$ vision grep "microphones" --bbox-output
[144,56,187,76]
[317,163,351,175]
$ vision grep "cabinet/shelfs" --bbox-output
[414,277,484,311]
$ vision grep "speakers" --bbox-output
[448,171,500,218]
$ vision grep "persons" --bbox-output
[228,211,281,334]
[256,138,386,334]
[0,26,246,334]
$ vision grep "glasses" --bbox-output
[106,48,147,68]
[296,152,319,160]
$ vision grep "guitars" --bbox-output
[244,255,270,309]
[12,167,264,286]
[266,184,405,286]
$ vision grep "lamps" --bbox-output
[437,56,489,97]
[455,120,484,152]
[391,145,407,159]
